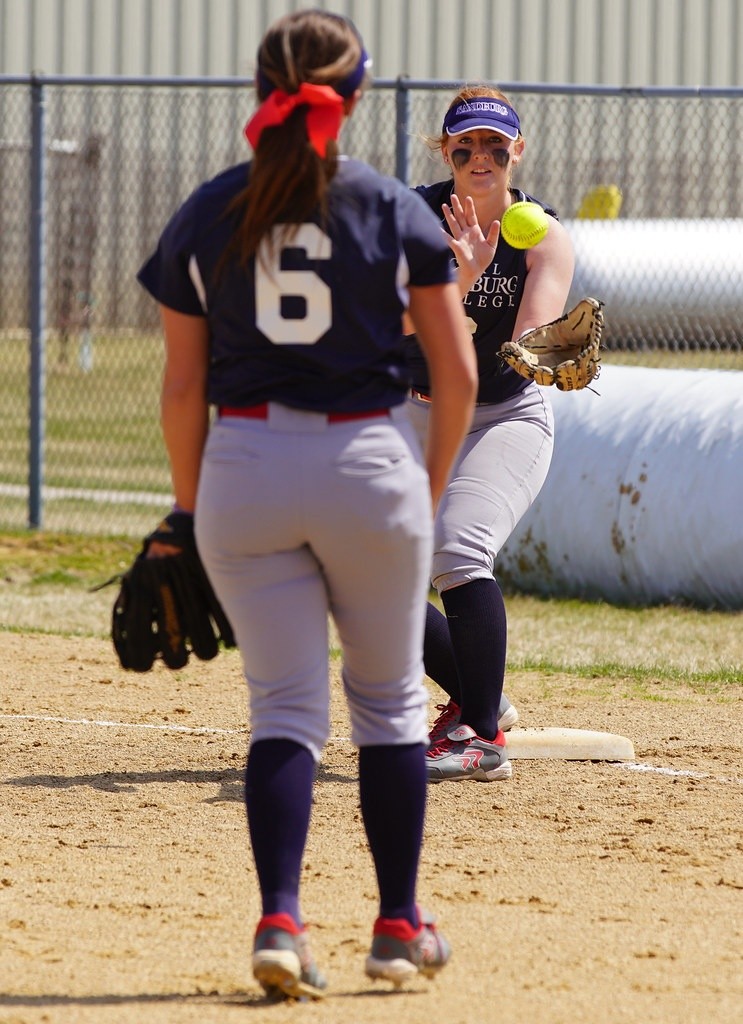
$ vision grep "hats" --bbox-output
[447,95,525,144]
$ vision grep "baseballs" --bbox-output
[500,200,549,251]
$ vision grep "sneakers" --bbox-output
[420,728,516,783]
[249,913,334,1001]
[363,915,449,994]
[430,691,522,740]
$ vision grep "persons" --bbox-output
[110,8,484,999]
[403,86,600,787]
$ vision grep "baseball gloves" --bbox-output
[108,508,240,674]
[498,296,607,393]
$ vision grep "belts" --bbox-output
[218,396,390,426]
[406,382,525,410]
